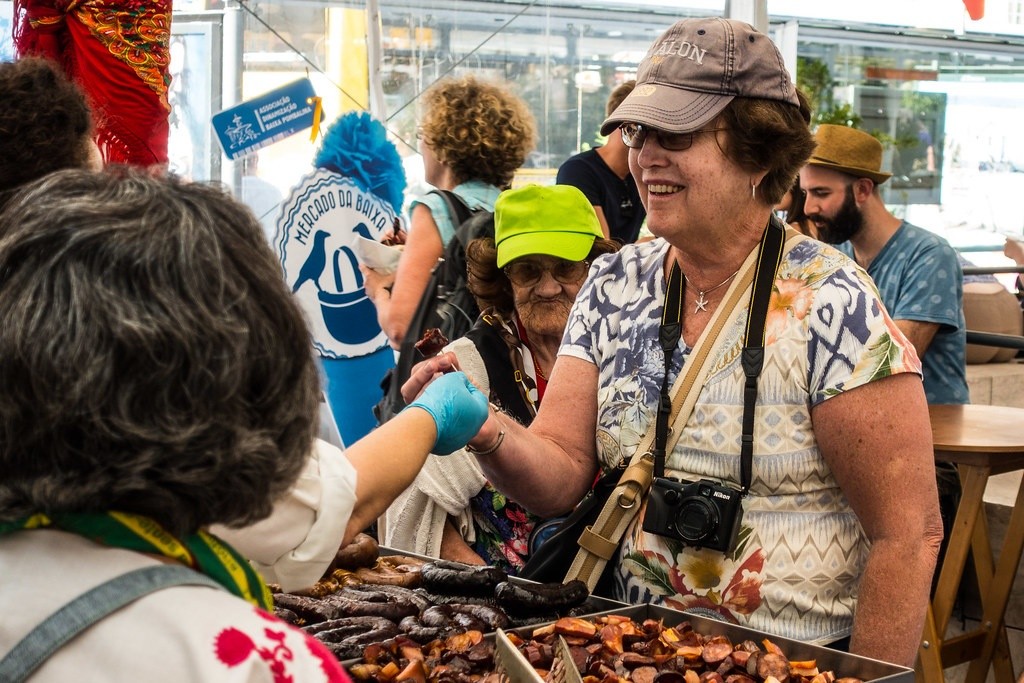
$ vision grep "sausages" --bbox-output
[414,329,447,357]
[267,532,593,661]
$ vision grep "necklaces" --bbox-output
[534,356,549,380]
[684,270,738,313]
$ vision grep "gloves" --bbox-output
[397,370,488,456]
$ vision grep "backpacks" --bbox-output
[371,189,495,427]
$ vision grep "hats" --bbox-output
[493,182,603,268]
[803,124,894,184]
[600,16,800,137]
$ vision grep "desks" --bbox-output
[915,403,1024,683]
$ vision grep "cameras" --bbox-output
[642,477,742,552]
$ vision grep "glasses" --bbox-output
[618,122,736,152]
[502,258,591,287]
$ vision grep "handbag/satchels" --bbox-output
[520,452,645,597]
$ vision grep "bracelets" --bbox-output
[466,402,505,456]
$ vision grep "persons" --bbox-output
[0,58,490,683]
[359,17,970,668]
